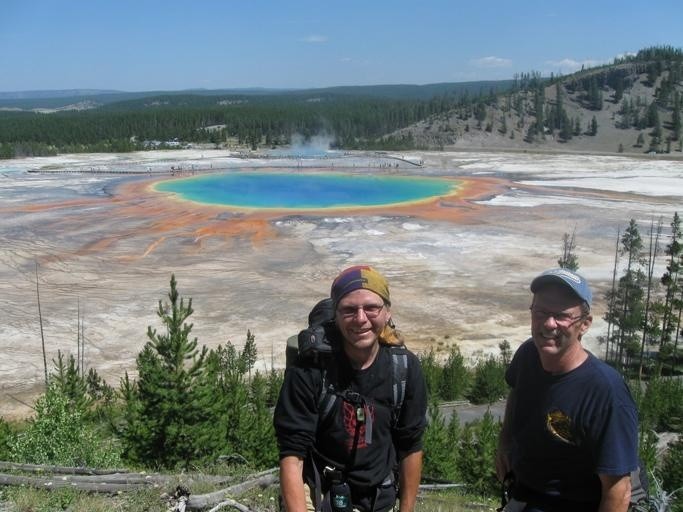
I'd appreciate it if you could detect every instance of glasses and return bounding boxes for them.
[530,305,584,327]
[336,303,385,319]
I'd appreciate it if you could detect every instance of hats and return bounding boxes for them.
[530,268,593,311]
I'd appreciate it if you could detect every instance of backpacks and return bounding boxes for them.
[285,298,410,436]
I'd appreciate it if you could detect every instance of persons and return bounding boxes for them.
[494,268,639,510]
[272,264,429,512]
